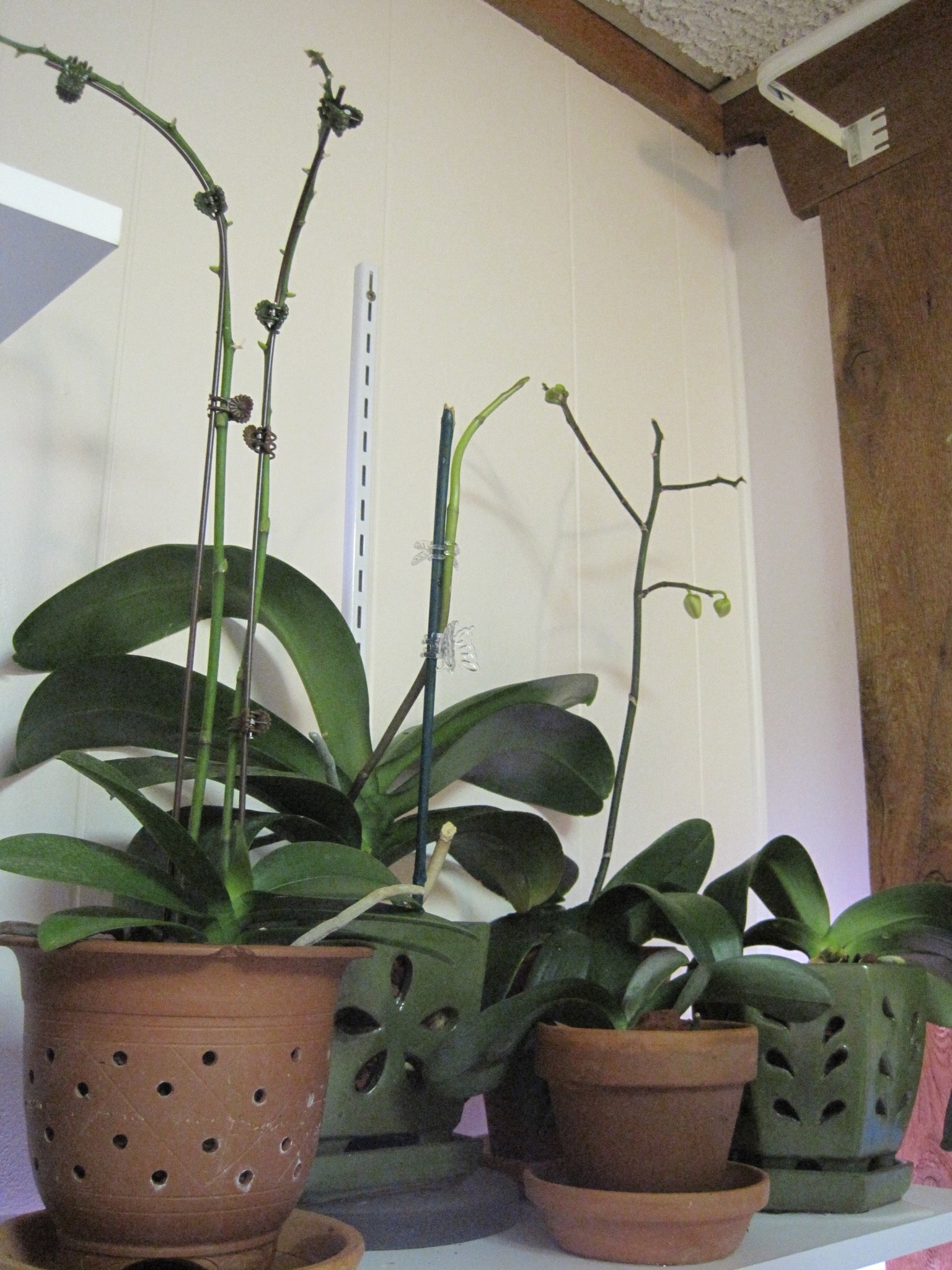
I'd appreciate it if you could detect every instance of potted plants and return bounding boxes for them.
[0,35,376,1269]
[17,378,621,1146]
[681,833,952,1213]
[468,392,750,1156]
[419,872,793,1264]
